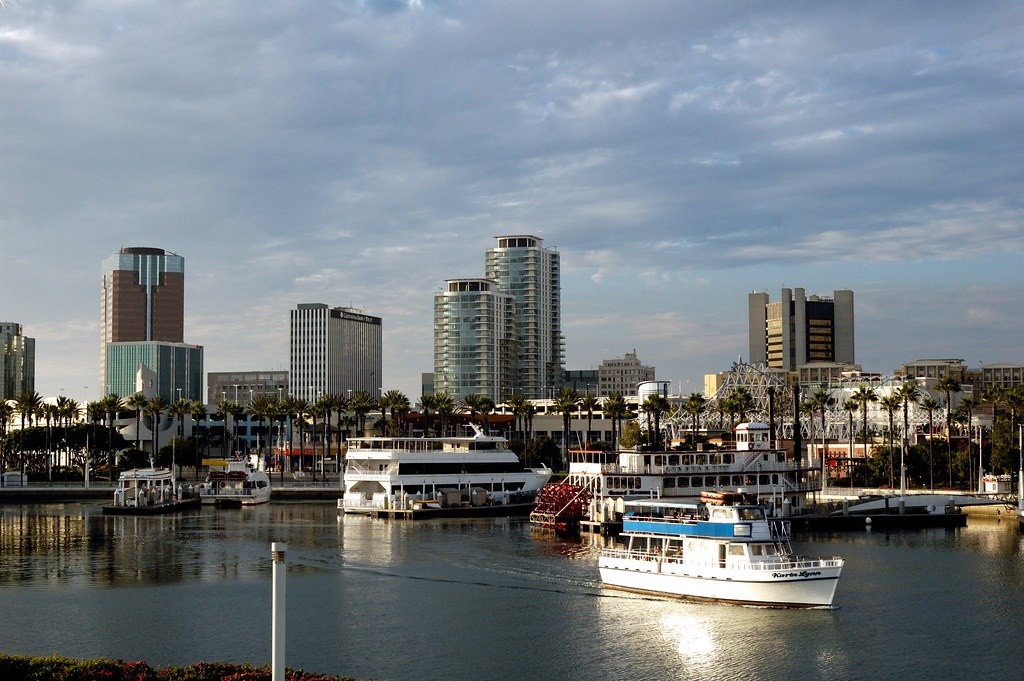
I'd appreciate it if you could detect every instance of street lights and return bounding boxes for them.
[60,379,681,412]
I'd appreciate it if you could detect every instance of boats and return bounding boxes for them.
[102,457,199,514]
[194,450,271,505]
[337,422,553,521]
[598,482,844,609]
[525,422,824,538]
